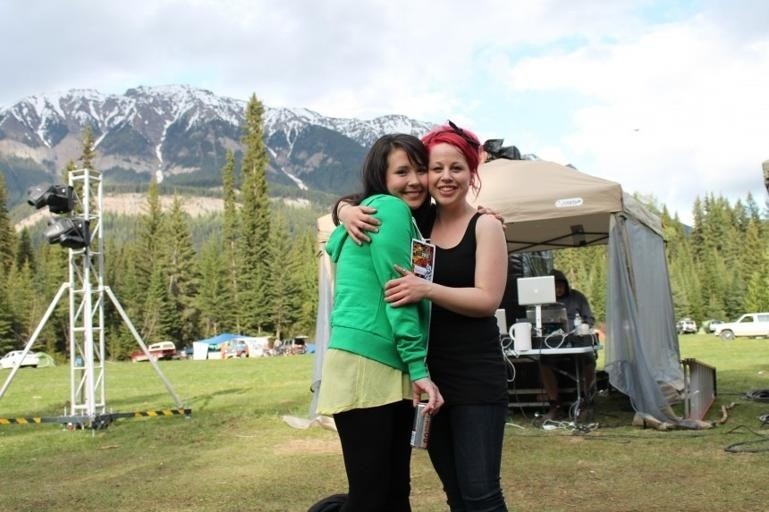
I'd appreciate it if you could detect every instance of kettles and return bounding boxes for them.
[509,321,533,352]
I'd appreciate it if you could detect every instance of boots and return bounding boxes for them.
[547,400,566,419]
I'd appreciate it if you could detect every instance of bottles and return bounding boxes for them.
[574,313,583,335]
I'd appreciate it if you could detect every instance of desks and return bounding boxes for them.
[503,344,604,423]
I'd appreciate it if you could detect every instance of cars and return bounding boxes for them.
[709,320,724,332]
[1,350,38,369]
[714,312,768,339]
[676,320,696,334]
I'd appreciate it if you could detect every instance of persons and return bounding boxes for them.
[539,271,599,420]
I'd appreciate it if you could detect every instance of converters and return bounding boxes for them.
[543,424,555,430]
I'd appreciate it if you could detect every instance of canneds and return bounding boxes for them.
[409,403,431,449]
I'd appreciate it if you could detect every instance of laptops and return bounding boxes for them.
[516,275,563,304]
[495,309,508,335]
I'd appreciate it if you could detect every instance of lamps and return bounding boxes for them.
[27,182,90,248]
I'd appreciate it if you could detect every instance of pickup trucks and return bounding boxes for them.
[134,339,177,362]
